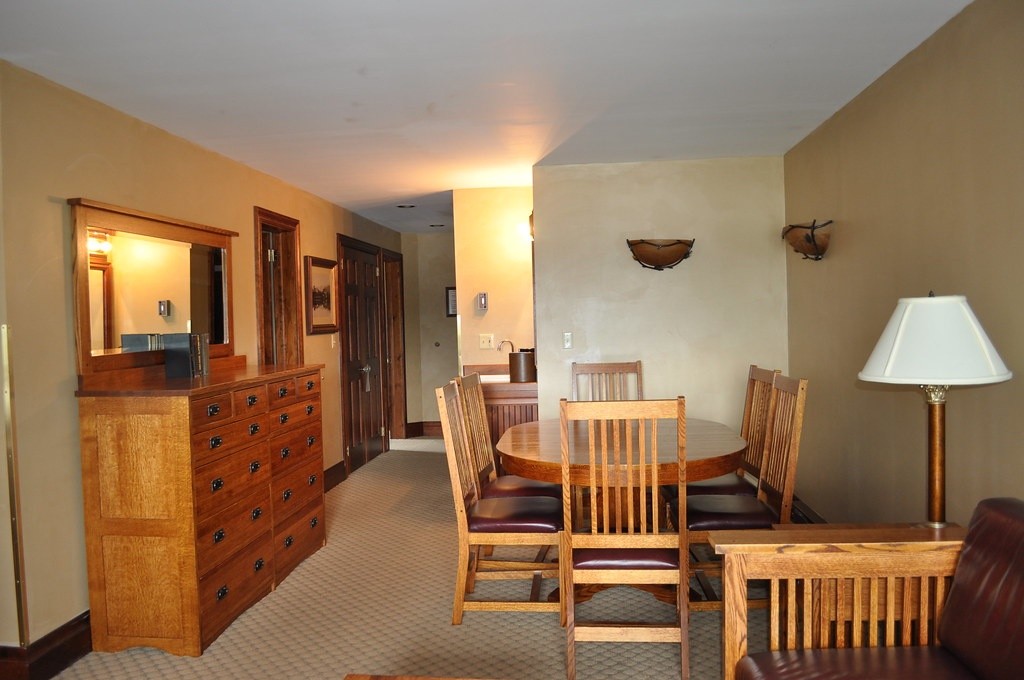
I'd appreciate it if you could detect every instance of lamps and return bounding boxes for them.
[781,218,833,261]
[856,290,1013,530]
[625,238,695,271]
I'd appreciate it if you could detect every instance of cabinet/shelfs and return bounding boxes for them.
[74,364,327,658]
[463,363,538,476]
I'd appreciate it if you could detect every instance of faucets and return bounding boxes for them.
[496,339,515,353]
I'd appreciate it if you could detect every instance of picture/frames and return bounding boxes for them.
[445,287,457,317]
[304,255,340,335]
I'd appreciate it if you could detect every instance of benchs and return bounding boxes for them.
[705,498,1024,680]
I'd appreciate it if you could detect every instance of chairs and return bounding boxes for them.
[660,365,809,644]
[572,360,644,531]
[435,370,573,627]
[559,396,690,680]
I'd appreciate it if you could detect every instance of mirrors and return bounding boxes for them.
[66,197,240,374]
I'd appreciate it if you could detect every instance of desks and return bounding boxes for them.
[772,518,966,651]
[495,418,749,606]
